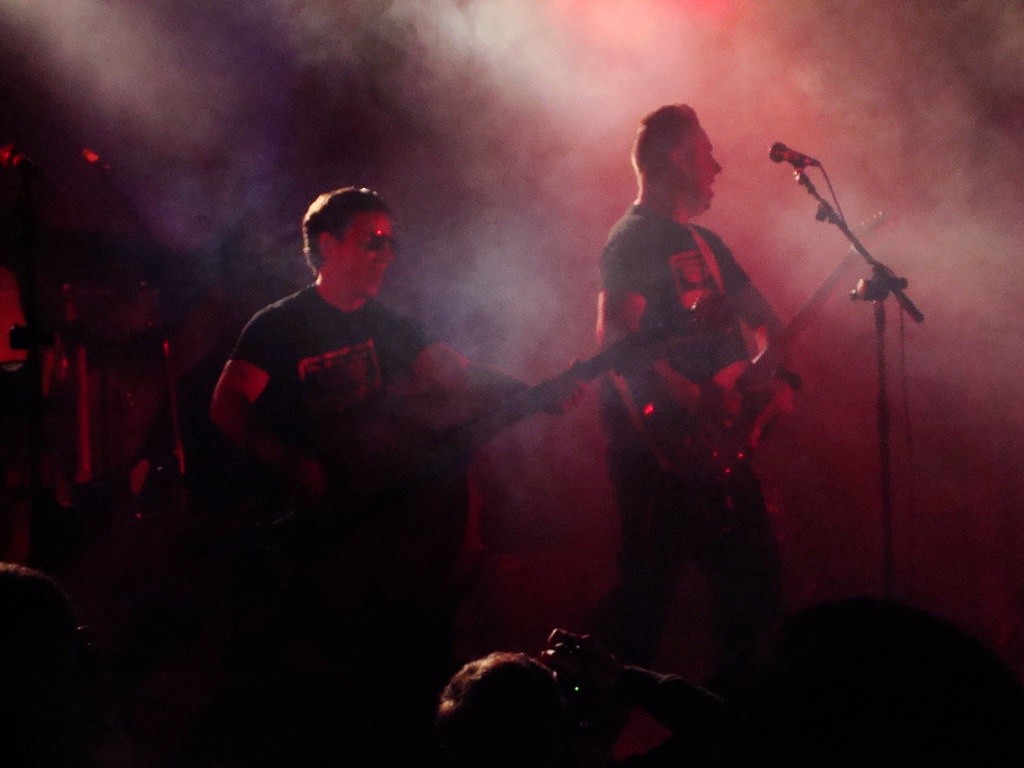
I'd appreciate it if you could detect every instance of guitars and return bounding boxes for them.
[693,209,886,453]
[275,319,710,600]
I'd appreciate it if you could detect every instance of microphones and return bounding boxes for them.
[769,142,821,166]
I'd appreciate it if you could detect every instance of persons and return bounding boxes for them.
[570,104,803,760]
[435,628,736,767]
[0,562,102,768]
[209,184,483,768]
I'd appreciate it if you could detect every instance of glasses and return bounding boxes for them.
[360,231,396,252]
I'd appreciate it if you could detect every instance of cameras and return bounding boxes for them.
[537,643,587,694]
